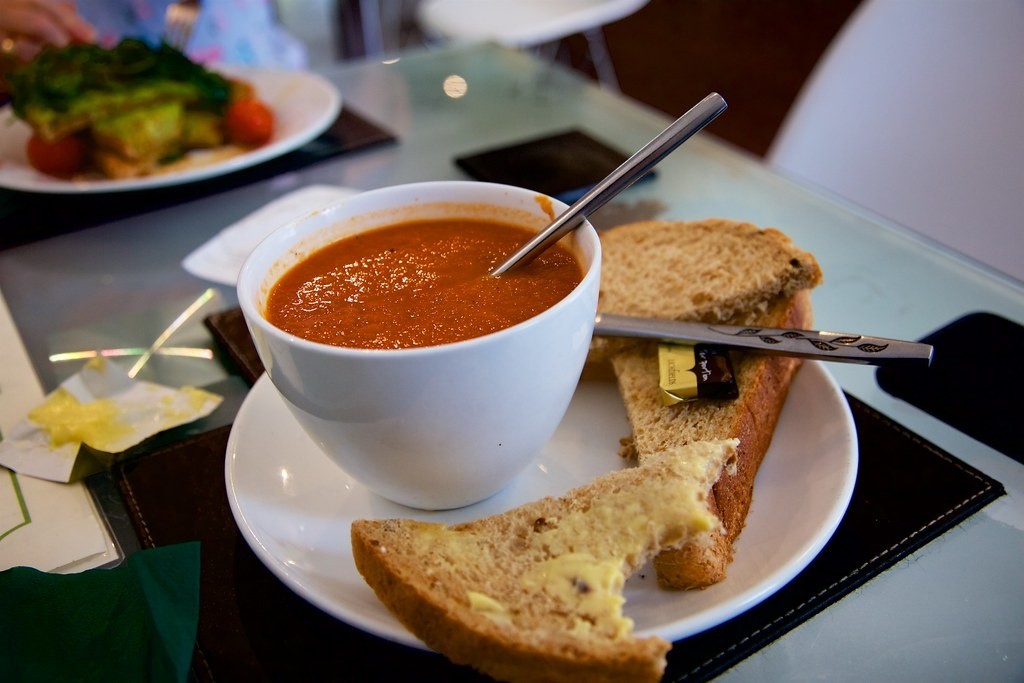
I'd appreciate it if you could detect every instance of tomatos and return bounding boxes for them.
[225,100,273,148]
[27,133,90,179]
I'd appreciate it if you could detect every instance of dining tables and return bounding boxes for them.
[0,38,1024,683]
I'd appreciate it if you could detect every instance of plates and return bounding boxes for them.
[225,357,860,652]
[0,68,344,191]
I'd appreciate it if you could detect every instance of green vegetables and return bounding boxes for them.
[6,34,233,124]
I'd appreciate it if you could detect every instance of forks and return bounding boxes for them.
[160,1,201,55]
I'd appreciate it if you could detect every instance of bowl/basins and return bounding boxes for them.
[236,182,601,510]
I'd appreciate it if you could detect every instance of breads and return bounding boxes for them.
[350,437,734,683]
[607,288,812,592]
[585,218,824,362]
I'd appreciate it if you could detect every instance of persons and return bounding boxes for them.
[0,1,309,106]
